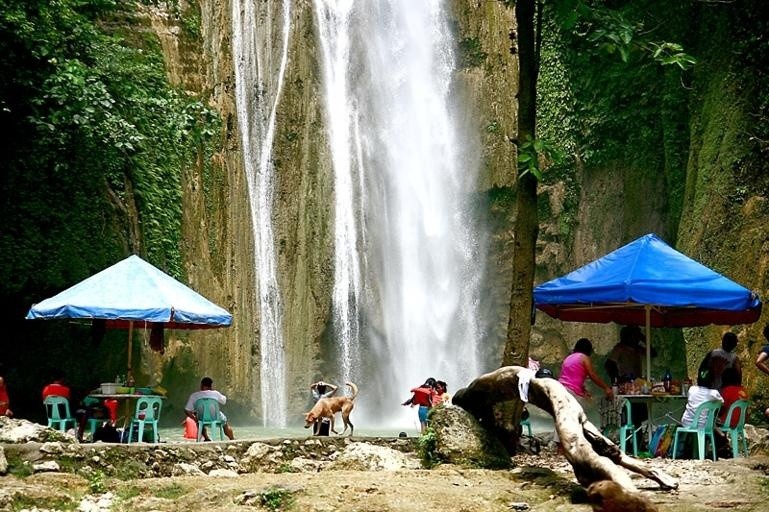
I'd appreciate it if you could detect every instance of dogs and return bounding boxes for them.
[302,382,358,436]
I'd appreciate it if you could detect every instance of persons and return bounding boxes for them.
[713,369,749,450]
[310,380,338,436]
[605,324,657,451]
[553,337,616,457]
[434,381,452,406]
[680,367,725,438]
[185,377,235,441]
[755,324,769,416]
[411,384,434,436]
[42,372,86,440]
[424,377,437,390]
[0,377,13,416]
[519,406,531,437]
[698,332,742,387]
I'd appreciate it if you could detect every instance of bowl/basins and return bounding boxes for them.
[117,386,135,394]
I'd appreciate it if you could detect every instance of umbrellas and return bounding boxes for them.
[532,235,763,451]
[24,253,232,429]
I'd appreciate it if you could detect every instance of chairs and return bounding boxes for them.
[81,393,170,445]
[44,395,79,438]
[716,397,754,460]
[598,395,641,457]
[194,396,232,445]
[672,399,726,462]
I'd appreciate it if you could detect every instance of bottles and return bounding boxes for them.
[612,375,663,394]
[664,368,672,392]
[115,375,135,388]
[674,384,681,392]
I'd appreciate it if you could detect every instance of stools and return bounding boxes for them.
[519,419,533,438]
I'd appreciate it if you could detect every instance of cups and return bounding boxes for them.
[682,385,688,395]
